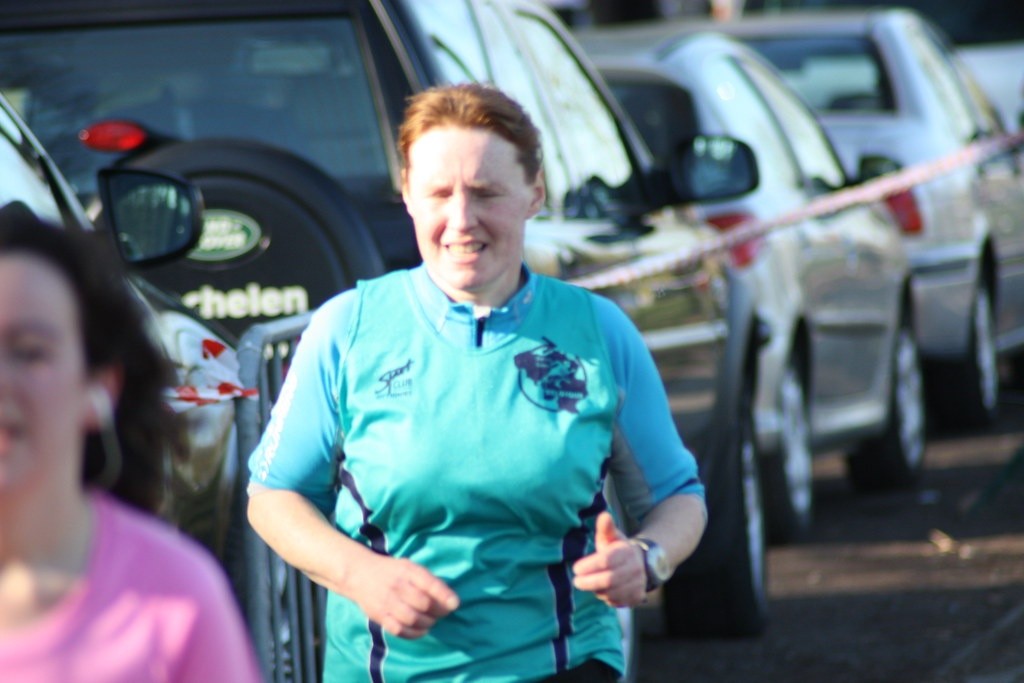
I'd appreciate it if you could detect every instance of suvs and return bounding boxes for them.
[0,0,774,683]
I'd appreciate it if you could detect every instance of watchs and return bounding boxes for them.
[632,538,672,592]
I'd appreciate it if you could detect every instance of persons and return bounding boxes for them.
[0,201,263,683]
[248,81,708,683]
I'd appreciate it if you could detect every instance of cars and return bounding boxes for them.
[578,9,1024,540]
